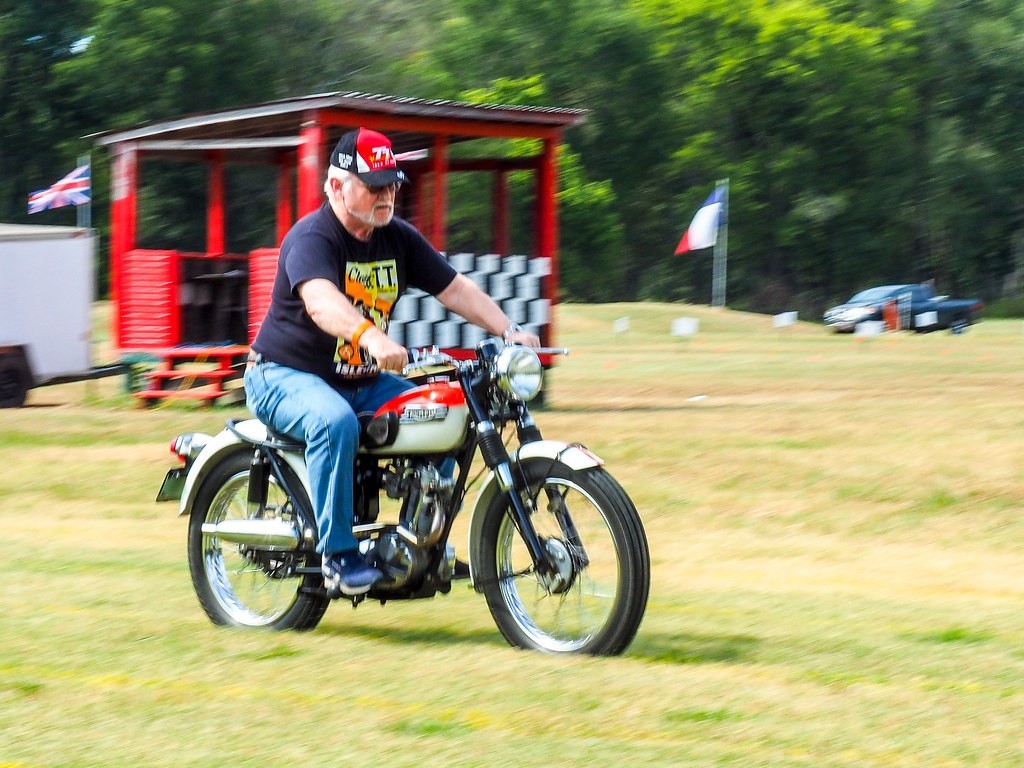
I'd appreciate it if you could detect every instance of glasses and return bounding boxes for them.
[365,180,401,195]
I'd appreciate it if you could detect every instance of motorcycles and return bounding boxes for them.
[154,334,652,658]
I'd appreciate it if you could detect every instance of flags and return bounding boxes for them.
[672,185,727,256]
[27,165,93,215]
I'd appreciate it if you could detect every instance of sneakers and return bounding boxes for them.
[321,552,383,595]
[451,558,468,583]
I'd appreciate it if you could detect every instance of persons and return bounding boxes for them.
[242,125,542,595]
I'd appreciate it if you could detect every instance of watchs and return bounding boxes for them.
[502,323,523,339]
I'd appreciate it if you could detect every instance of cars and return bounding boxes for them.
[821,283,984,333]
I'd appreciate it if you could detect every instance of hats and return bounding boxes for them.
[330,126,409,186]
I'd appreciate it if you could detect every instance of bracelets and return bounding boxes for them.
[337,319,376,366]
